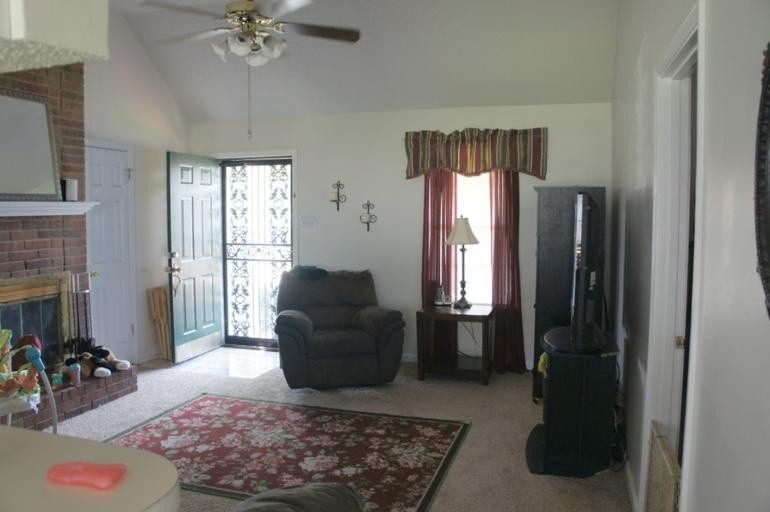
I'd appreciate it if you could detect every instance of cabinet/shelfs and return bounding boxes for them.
[525,185,619,478]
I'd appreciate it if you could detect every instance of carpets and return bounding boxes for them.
[99,391,472,512]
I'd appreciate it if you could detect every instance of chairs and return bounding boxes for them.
[0,341,40,426]
[274,269,406,388]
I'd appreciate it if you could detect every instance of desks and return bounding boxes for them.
[0,425,179,512]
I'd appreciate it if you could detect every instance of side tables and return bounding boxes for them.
[416,303,496,386]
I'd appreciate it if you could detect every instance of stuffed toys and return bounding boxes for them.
[7,336,130,393]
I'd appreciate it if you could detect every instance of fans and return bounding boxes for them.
[138,0,360,48]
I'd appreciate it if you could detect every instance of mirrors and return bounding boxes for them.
[0,87,63,201]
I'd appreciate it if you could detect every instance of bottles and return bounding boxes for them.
[69,363,80,385]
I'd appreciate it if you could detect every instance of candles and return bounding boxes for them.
[361,213,370,222]
[329,190,337,200]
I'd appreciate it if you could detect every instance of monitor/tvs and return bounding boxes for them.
[562,190,609,355]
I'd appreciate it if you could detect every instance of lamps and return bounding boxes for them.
[213,33,287,68]
[445,215,479,308]
